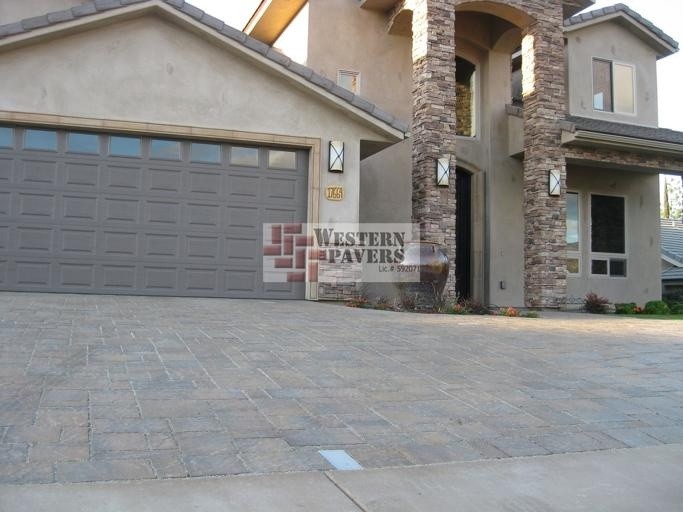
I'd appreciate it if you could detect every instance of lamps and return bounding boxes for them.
[549,169,561,196]
[437,158,450,186]
[329,141,345,172]
